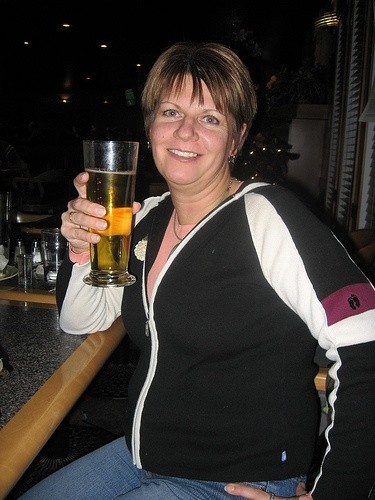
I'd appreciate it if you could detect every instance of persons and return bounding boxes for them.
[7,39,375,500]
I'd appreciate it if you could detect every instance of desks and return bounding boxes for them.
[0,201,127,500]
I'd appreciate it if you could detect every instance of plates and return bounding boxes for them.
[0,265,18,281]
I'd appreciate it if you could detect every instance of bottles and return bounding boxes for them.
[30,238,42,267]
[14,239,26,264]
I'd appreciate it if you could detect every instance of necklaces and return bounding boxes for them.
[173,174,237,240]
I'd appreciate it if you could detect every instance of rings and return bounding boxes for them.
[68,211,79,224]
[267,491,275,499]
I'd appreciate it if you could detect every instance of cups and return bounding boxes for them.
[17,254,33,292]
[82,139,140,287]
[41,228,69,287]
